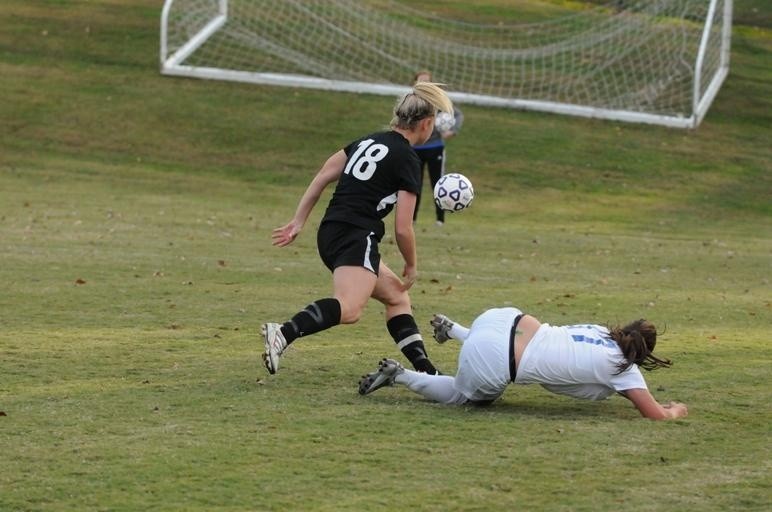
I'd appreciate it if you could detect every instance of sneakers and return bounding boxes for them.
[359,358,404,395]
[262,323,287,374]
[430,314,454,344]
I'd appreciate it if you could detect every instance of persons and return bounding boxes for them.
[358,307,688,419]
[412,70,465,224]
[260,81,441,376]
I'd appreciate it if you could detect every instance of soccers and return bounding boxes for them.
[434,112,456,133]
[434,173,474,212]
